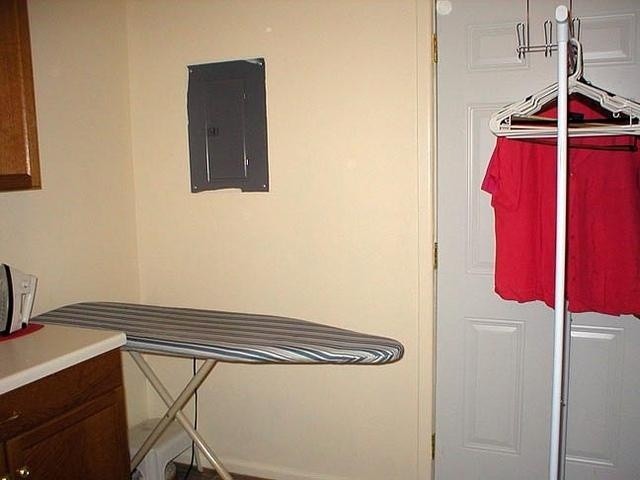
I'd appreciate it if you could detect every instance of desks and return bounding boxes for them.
[25,302,404,479]
[1,322,130,479]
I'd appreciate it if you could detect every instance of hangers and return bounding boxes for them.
[488,36,639,142]
